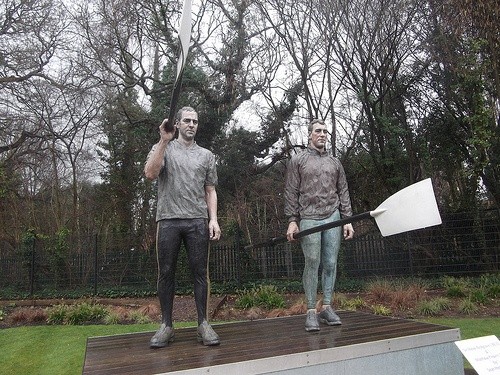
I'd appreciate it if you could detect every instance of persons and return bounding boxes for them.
[144,107,222,348]
[283,120,355,332]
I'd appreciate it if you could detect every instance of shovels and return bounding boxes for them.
[245,177,442,252]
[163,0,192,133]
